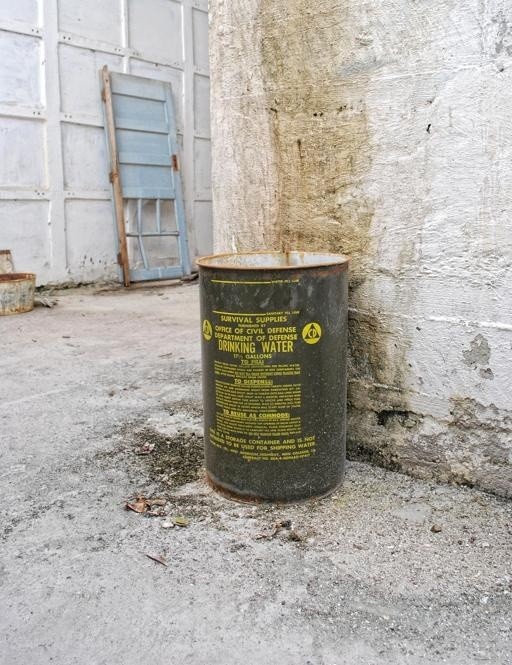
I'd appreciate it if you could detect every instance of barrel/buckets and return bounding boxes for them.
[194,249,351,506]
